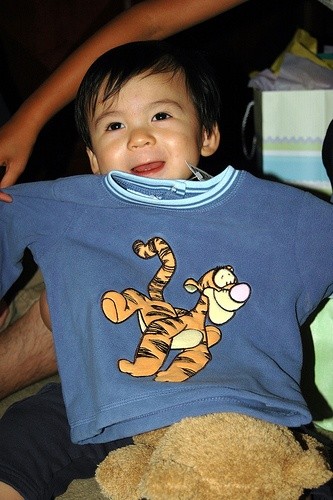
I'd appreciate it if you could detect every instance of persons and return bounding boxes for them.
[1,290,57,397]
[1,40,333,500]
[0,0,246,204]
